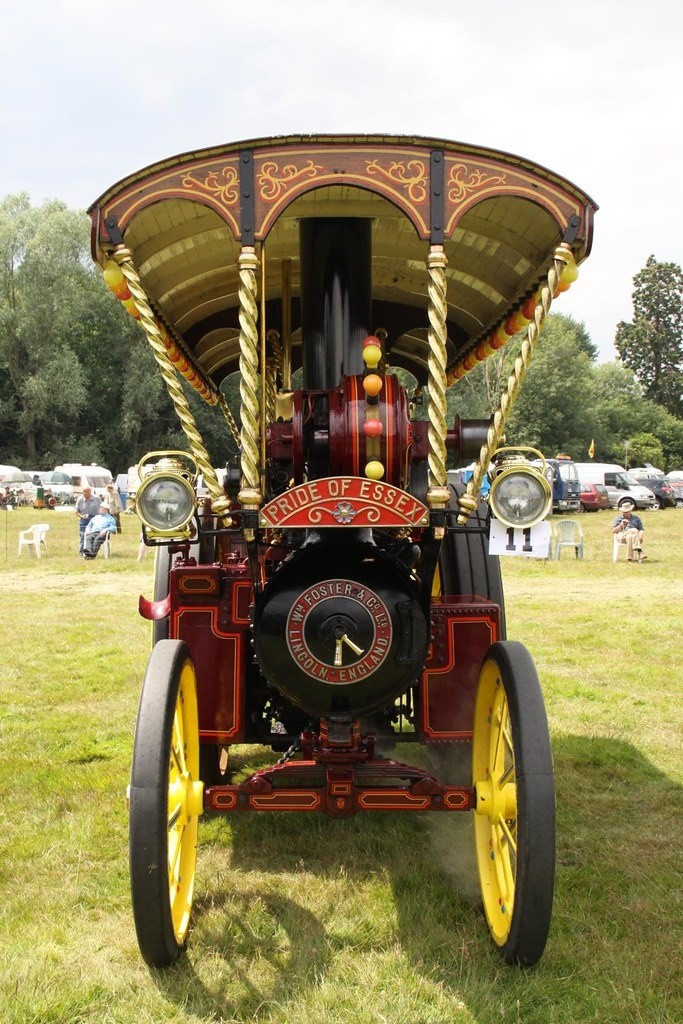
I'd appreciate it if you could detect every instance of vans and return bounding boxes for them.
[127,463,154,499]
[627,468,664,479]
[114,473,128,494]
[547,463,656,512]
[54,463,115,501]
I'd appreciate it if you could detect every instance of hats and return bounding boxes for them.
[619,502,634,513]
[99,502,110,510]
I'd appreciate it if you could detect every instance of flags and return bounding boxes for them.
[588,438,595,458]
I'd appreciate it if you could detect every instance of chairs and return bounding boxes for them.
[613,533,641,563]
[83,531,112,559]
[18,524,51,559]
[555,520,585,560]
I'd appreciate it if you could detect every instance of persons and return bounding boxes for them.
[124,499,137,514]
[107,485,124,534]
[3,487,26,507]
[83,502,117,560]
[612,502,647,563]
[75,487,103,558]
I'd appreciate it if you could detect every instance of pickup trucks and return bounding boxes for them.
[22,471,75,505]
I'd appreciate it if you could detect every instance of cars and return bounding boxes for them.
[574,483,610,514]
[534,458,580,514]
[663,471,683,499]
[84,132,602,967]
[635,479,676,510]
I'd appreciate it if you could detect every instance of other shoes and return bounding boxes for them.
[639,555,647,560]
[83,549,96,560]
[628,559,631,563]
